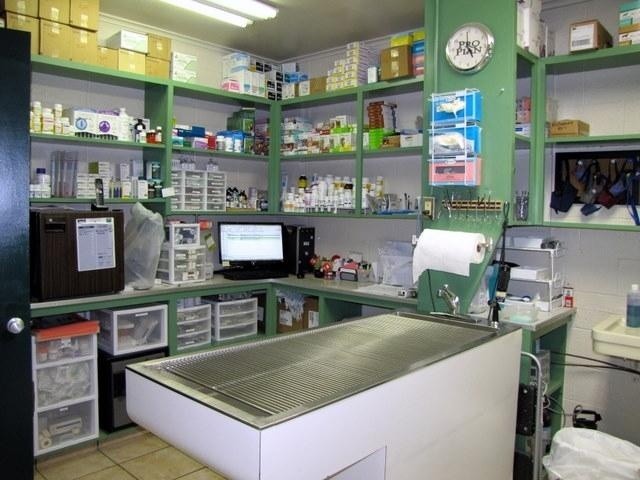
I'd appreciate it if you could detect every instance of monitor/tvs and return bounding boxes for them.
[219,222,287,280]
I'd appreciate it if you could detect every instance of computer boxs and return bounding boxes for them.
[283,223,315,275]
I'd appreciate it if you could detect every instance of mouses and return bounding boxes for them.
[297,273,305,280]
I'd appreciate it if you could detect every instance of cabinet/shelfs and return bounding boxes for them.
[29,43,639,480]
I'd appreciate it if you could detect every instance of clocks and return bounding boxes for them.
[443,22,495,75]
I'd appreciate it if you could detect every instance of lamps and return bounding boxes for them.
[161,0,280,28]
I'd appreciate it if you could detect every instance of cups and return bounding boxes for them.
[516,189,531,222]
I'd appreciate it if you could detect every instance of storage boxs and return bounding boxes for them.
[0,0,171,79]
[568,18,614,55]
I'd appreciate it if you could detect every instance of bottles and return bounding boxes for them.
[119,107,128,116]
[215,135,242,152]
[285,173,384,212]
[135,117,162,143]
[30,168,51,199]
[627,283,640,327]
